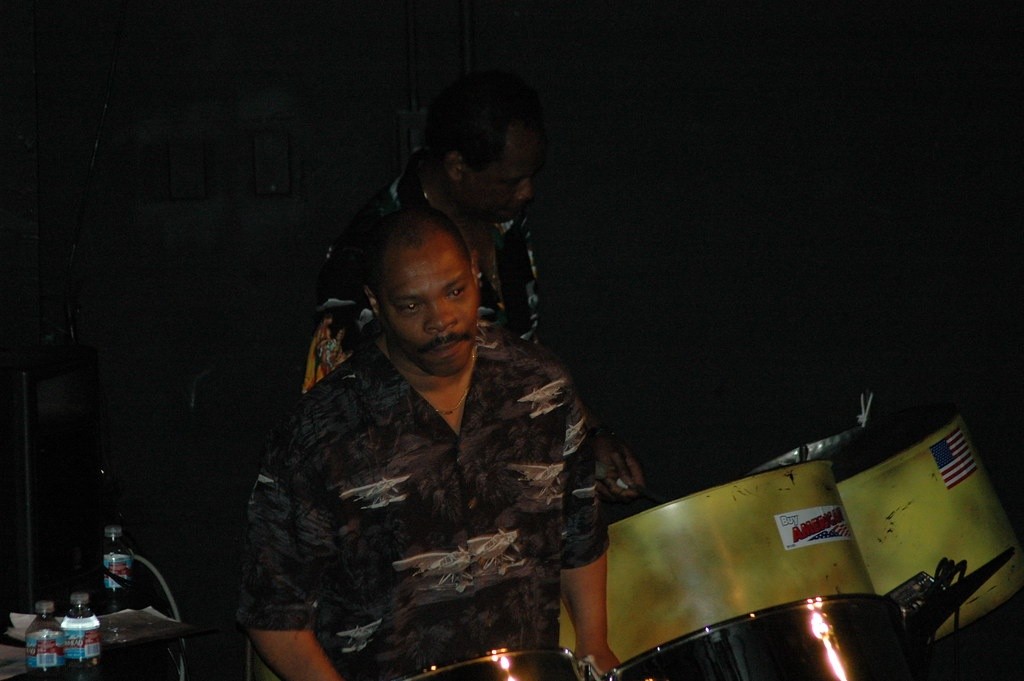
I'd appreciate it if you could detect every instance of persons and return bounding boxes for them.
[235,201,624,681]
[295,67,652,503]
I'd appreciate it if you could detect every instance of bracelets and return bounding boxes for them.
[584,421,617,443]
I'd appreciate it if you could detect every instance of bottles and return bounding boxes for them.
[101,523,134,613]
[59,592,101,681]
[23,601,64,681]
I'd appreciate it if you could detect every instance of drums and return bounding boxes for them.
[554,456,876,664]
[809,405,1022,644]
[611,593,928,679]
[403,647,583,680]
